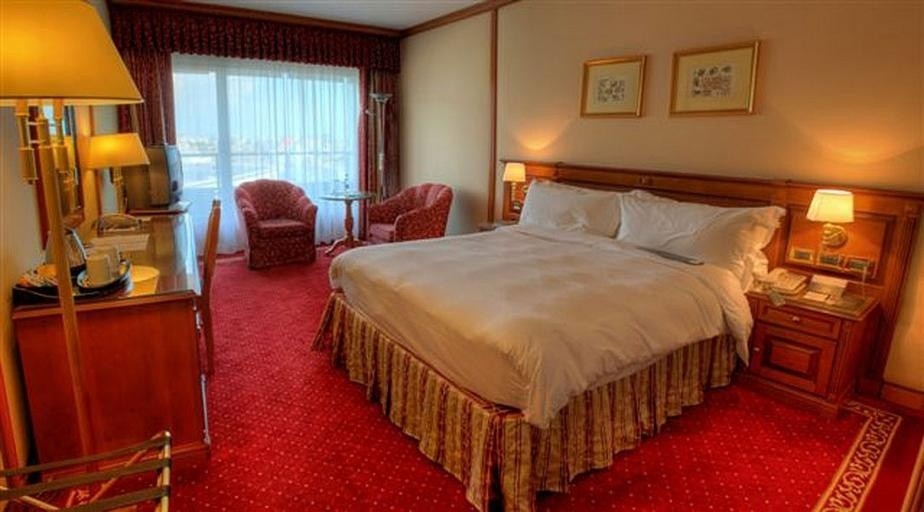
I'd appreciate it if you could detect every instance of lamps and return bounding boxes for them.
[804,188,853,250]
[499,159,525,212]
[83,130,148,230]
[3,0,148,509]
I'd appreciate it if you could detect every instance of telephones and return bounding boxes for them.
[763,267,808,295]
[104,212,137,228]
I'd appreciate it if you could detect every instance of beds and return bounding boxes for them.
[296,159,783,512]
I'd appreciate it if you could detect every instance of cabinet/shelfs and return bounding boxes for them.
[364,88,391,200]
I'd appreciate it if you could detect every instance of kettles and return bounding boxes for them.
[44,228,89,276]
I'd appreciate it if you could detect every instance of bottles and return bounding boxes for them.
[343,173,350,194]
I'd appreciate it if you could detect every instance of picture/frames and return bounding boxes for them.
[666,38,760,119]
[580,53,646,119]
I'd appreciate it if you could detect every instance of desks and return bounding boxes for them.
[13,212,209,491]
[320,193,373,254]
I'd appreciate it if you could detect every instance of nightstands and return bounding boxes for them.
[745,279,881,418]
[477,220,516,231]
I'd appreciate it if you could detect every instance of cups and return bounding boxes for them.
[96,245,120,271]
[85,254,110,285]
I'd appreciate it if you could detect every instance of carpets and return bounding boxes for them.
[4,236,924,512]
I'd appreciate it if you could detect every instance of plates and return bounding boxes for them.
[16,281,59,296]
[76,260,130,291]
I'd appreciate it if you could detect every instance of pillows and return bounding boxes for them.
[617,190,788,276]
[515,180,620,241]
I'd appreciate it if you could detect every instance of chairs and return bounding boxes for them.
[234,176,318,269]
[200,197,227,379]
[366,184,452,245]
